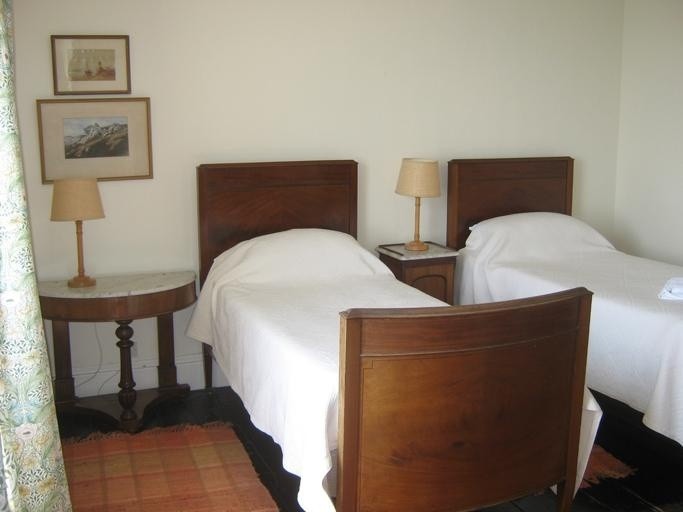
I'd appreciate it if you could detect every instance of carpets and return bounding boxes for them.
[580,444,635,487]
[62,421,280,511]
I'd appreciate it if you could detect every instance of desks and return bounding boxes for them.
[38,271,198,434]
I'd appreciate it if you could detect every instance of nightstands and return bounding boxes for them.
[375,241,459,305]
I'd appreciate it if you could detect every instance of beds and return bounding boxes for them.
[447,156,682,445]
[186,160,604,512]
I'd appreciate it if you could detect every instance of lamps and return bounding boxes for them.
[395,158,441,251]
[50,177,106,288]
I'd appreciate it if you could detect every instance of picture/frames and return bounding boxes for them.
[51,35,131,95]
[36,98,154,185]
